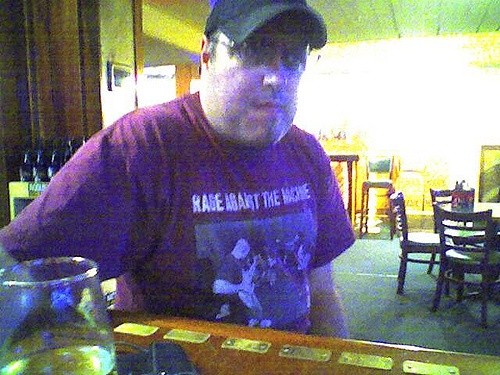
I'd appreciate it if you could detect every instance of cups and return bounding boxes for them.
[0,257,118,375]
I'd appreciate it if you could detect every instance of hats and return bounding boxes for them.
[205,1,326,49]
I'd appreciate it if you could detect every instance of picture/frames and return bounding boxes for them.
[108,63,134,93]
[478,144,500,203]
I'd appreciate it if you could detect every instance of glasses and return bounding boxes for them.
[217,43,306,75]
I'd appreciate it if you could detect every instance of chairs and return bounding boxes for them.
[427,189,460,274]
[390,192,452,296]
[433,204,500,328]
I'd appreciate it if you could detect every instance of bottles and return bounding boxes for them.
[18,135,89,182]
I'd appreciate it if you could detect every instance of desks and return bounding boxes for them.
[329,155,360,227]
[1,309,500,375]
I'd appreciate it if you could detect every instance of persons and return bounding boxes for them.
[0,0,358,339]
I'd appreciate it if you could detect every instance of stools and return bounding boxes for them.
[360,182,397,240]
